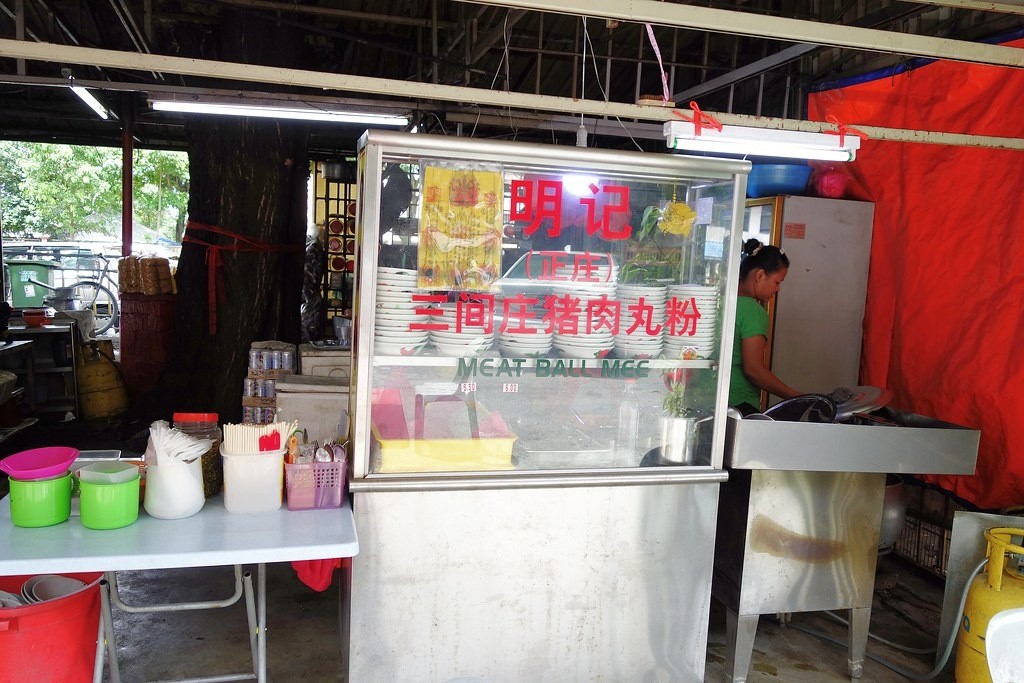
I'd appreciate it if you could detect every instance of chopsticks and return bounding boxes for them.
[223,420,300,453]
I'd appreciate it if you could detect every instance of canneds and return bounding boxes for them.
[243,350,293,427]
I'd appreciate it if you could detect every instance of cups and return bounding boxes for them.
[336,325,351,346]
[145,456,205,520]
[8,469,73,528]
[79,469,143,530]
[220,441,288,514]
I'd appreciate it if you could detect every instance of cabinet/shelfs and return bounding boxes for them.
[7,319,81,420]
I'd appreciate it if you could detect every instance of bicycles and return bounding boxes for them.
[69,250,123,335]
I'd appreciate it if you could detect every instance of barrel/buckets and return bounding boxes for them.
[657,415,714,465]
[0,572,112,683]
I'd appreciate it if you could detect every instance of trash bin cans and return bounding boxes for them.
[3,259,61,307]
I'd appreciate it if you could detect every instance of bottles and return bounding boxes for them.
[172,412,222,498]
[617,377,641,465]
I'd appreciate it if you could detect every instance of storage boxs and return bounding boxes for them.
[274,374,350,445]
[370,400,517,473]
[0,386,25,428]
[297,343,351,377]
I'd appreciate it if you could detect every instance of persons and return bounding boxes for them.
[728,238,804,418]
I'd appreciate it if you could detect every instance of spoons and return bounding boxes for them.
[316,443,346,508]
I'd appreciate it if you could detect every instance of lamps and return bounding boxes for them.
[660,121,861,163]
[146,91,413,126]
[60,68,110,120]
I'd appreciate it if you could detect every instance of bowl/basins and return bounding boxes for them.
[664,284,718,360]
[374,266,430,356]
[22,309,55,326]
[429,302,496,357]
[497,320,552,358]
[0,575,88,608]
[553,263,620,358]
[615,282,668,359]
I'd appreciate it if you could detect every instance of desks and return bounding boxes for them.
[0,457,359,683]
